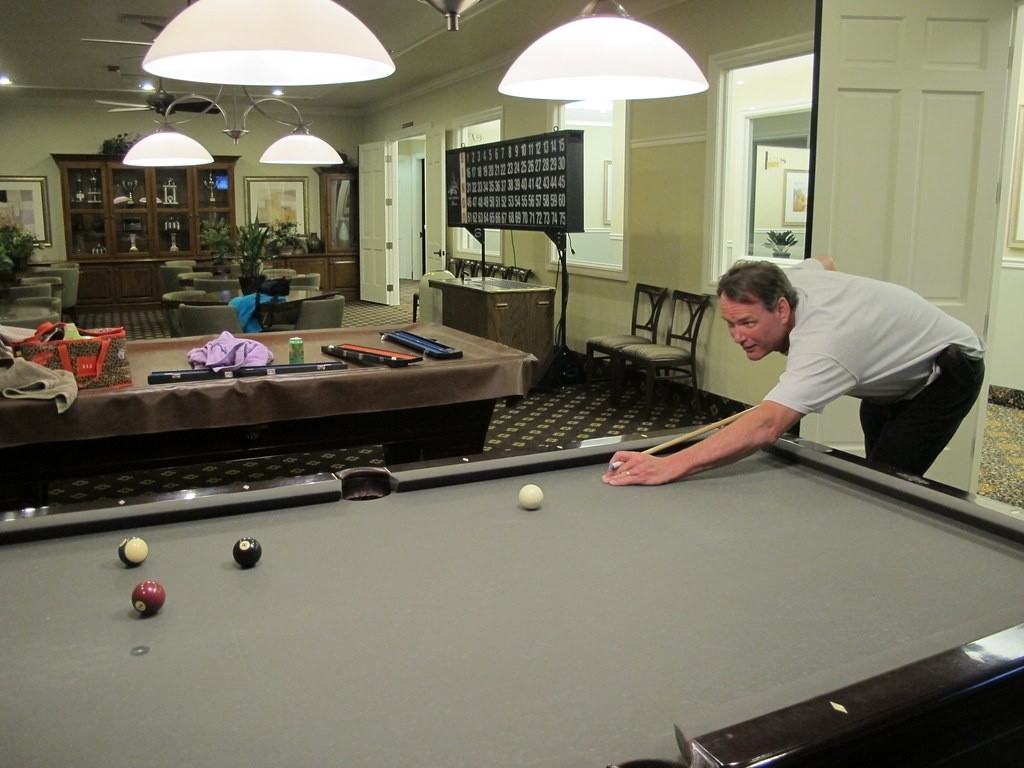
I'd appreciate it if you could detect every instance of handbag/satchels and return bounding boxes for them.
[12,320,132,391]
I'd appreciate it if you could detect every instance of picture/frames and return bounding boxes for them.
[0,176,53,248]
[242,175,310,238]
[780,169,810,226]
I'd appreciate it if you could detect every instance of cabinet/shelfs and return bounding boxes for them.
[278,252,332,291]
[49,153,242,311]
[312,167,360,296]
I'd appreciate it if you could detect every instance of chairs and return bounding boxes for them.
[584,283,669,406]
[0,262,81,330]
[155,260,346,338]
[619,289,712,418]
[413,258,532,322]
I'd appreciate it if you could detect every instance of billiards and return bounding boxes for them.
[232,537,261,566]
[518,484,544,509]
[131,581,165,615]
[118,536,150,566]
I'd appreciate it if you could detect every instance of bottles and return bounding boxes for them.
[63,323,81,339]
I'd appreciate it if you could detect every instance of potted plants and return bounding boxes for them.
[0,241,17,285]
[761,230,799,259]
[199,215,235,276]
[234,206,272,297]
[0,222,46,272]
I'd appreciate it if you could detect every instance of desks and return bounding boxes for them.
[162,290,341,328]
[427,275,556,390]
[0,421,1024,768]
[0,319,538,508]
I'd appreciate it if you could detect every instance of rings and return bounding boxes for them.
[624,471,631,476]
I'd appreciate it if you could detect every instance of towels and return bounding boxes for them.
[227,292,287,332]
[187,331,274,373]
[0,340,79,414]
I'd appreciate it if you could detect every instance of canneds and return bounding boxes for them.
[288,336,305,364]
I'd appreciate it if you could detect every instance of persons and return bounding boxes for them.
[602,258,986,486]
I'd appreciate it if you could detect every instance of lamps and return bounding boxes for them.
[241,96,344,165]
[119,93,228,169]
[419,0,480,32]
[140,0,396,88]
[497,0,710,103]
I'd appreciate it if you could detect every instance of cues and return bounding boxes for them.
[609,404,761,471]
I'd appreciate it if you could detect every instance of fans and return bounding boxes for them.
[92,76,222,116]
